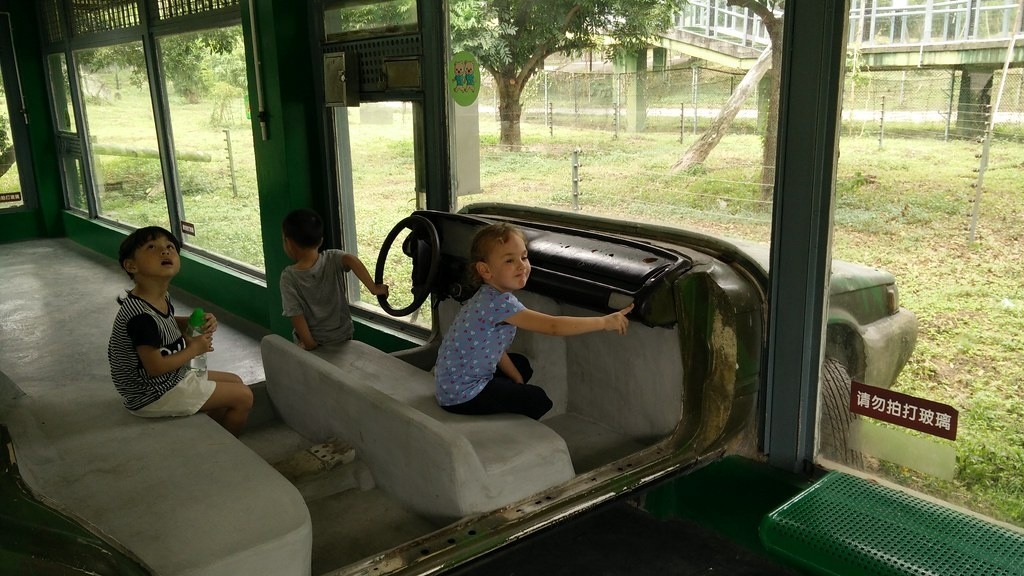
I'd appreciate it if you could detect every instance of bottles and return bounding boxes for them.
[184,308,208,377]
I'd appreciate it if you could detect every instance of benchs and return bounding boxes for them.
[0,371,313,576]
[260,334,576,530]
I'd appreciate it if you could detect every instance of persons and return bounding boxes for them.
[434,222,634,421]
[279,208,389,351]
[107,226,253,439]
